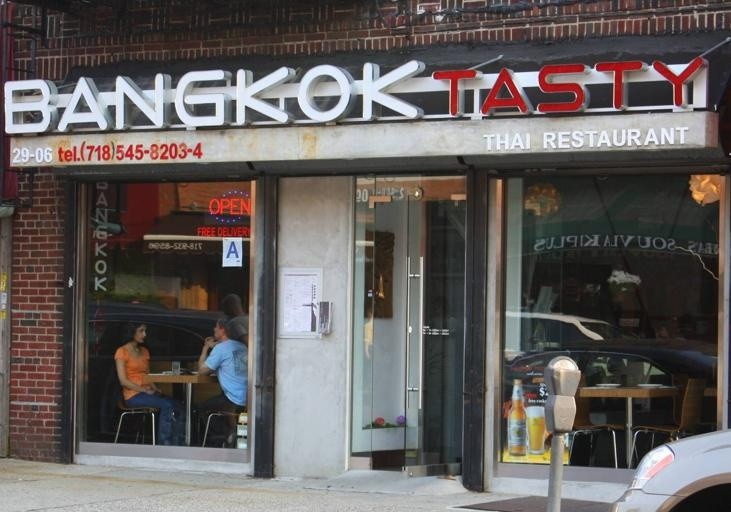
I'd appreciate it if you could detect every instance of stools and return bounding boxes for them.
[199,411,239,447]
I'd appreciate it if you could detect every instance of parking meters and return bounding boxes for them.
[539,356,581,511]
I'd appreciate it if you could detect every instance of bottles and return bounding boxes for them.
[507,378,528,455]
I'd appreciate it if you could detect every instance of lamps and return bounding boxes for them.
[690,175,722,207]
[526,184,562,219]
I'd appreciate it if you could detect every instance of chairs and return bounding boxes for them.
[567,375,641,469]
[625,378,705,471]
[113,374,158,445]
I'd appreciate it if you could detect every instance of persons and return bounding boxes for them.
[114,317,185,446]
[195,317,248,449]
[221,293,248,346]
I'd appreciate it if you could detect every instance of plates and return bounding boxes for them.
[596,383,621,387]
[638,384,663,388]
[192,372,199,374]
[163,371,183,374]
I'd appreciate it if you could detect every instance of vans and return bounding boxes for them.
[445,307,635,386]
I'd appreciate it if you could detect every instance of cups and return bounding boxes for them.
[171,361,180,376]
[525,406,550,455]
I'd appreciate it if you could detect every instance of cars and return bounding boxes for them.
[601,424,731,512]
[498,339,717,454]
[88,301,164,314]
[90,310,233,438]
